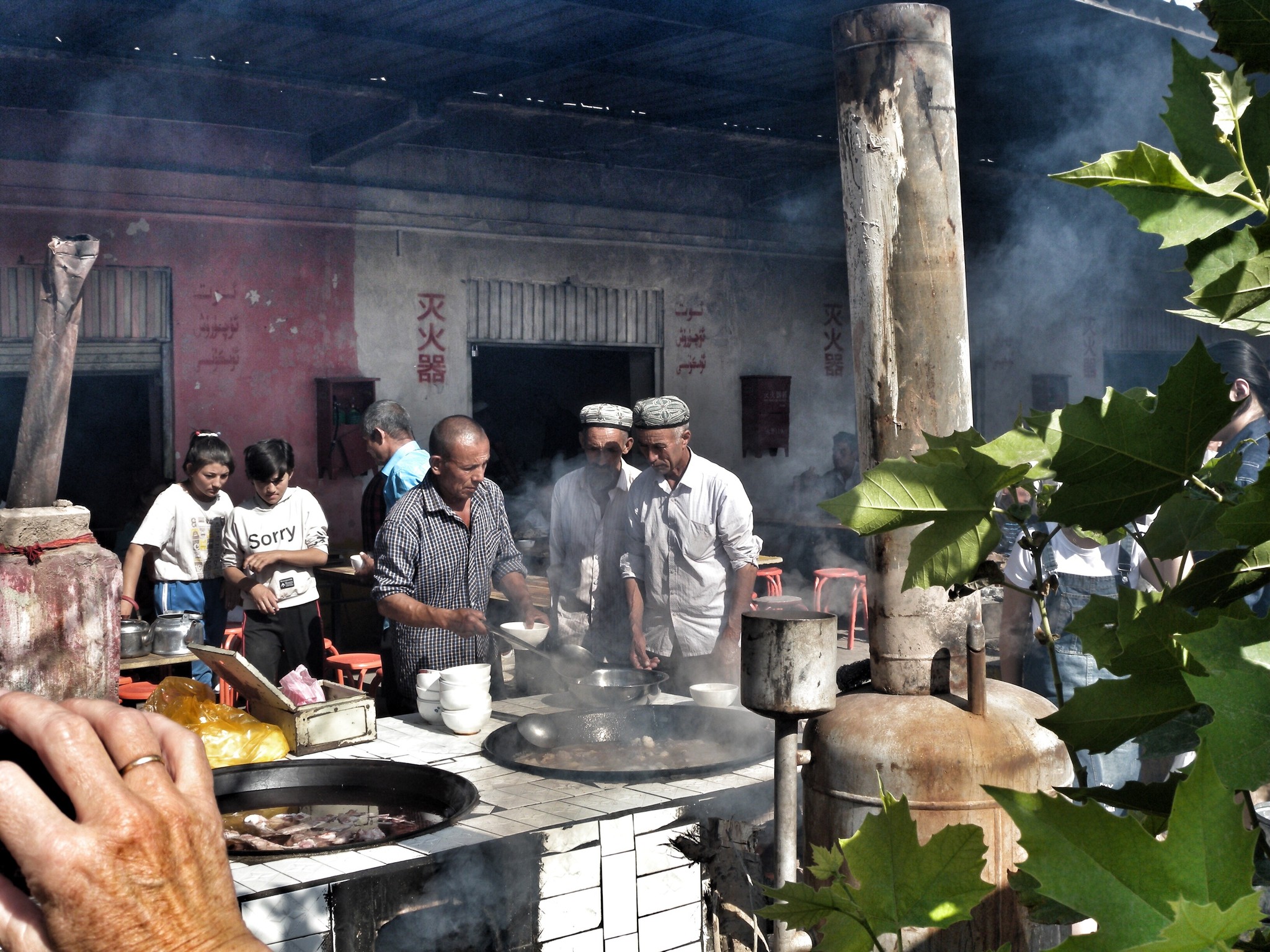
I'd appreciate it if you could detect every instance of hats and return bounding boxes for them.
[633,394,691,430]
[580,402,633,432]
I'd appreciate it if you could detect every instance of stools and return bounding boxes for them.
[813,568,861,615]
[749,590,759,612]
[324,651,384,692]
[324,638,355,686]
[846,574,869,649]
[751,594,809,612]
[366,668,385,704]
[217,621,245,706]
[756,565,784,610]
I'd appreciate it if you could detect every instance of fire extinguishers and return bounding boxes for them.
[347,402,361,424]
[329,395,346,455]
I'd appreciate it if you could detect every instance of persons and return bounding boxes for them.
[1147,339,1270,630]
[372,413,552,720]
[620,395,759,707]
[995,469,1196,817]
[115,428,235,691]
[0,689,274,951]
[359,398,436,696]
[516,483,557,537]
[546,403,644,669]
[222,437,331,708]
[549,436,587,481]
[995,485,1040,556]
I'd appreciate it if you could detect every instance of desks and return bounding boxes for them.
[757,553,784,565]
[315,564,549,704]
[751,508,852,530]
[118,645,203,687]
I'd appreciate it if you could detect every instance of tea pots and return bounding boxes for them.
[119,595,157,659]
[150,610,204,656]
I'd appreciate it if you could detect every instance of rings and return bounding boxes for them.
[363,556,371,560]
[118,754,165,777]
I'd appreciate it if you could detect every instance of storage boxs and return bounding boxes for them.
[188,642,378,758]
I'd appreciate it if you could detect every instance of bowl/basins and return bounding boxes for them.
[518,539,535,550]
[350,554,370,571]
[499,621,550,650]
[689,683,739,707]
[557,666,670,708]
[415,663,493,735]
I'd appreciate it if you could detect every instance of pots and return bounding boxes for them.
[484,703,776,782]
[211,758,479,866]
